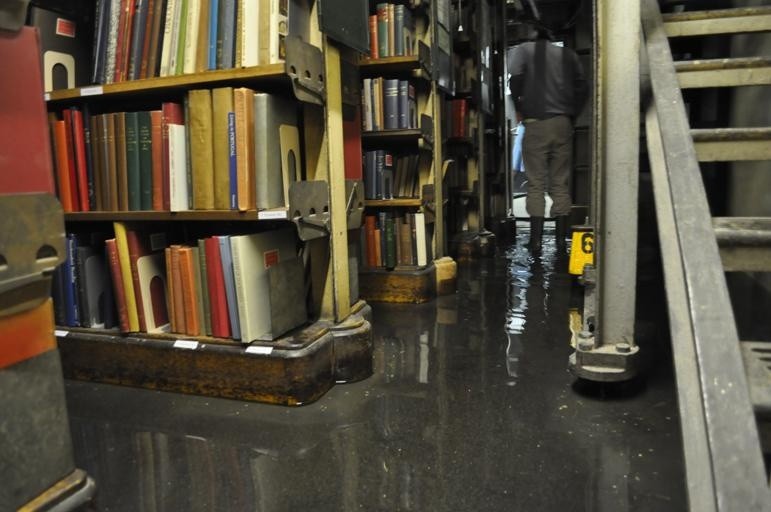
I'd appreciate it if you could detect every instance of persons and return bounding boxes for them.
[518,251,577,421]
[502,20,591,258]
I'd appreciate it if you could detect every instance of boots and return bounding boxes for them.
[555,215,568,247]
[523,218,544,252]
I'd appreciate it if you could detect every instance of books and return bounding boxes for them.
[368,2,414,59]
[360,150,420,199]
[51,86,297,212]
[362,77,418,131]
[54,221,296,343]
[363,210,427,269]
[437,6,479,230]
[90,0,289,85]
